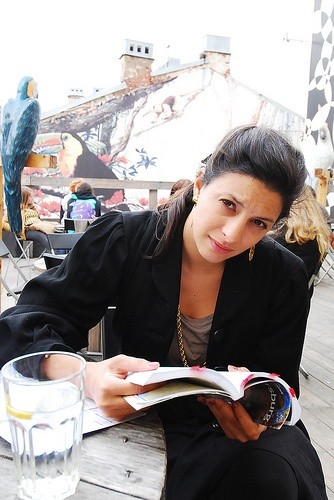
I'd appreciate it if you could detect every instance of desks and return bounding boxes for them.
[0,405,167,500]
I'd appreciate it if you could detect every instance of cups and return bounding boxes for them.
[1,351,86,500]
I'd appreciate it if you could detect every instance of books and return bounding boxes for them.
[125,363,303,431]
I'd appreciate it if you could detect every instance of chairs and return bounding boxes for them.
[0,218,94,304]
[298,242,334,379]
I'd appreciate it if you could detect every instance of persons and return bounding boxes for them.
[55,177,103,240]
[0,126,327,500]
[170,178,192,196]
[1,187,54,258]
[265,179,333,300]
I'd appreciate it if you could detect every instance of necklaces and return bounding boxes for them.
[177,303,208,368]
